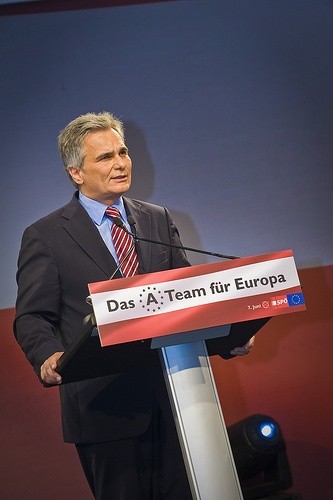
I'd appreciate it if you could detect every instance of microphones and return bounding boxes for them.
[108,216,139,279]
[114,217,239,259]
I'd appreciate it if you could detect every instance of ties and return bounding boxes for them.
[105,206,142,279]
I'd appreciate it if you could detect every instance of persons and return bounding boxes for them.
[12,109,256,500]
